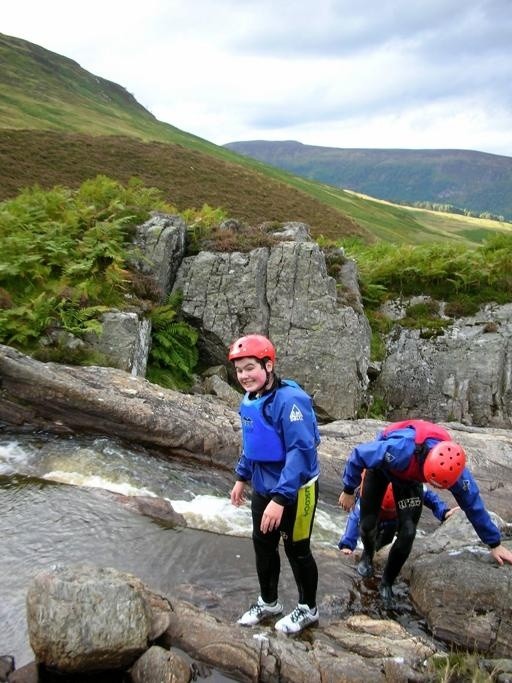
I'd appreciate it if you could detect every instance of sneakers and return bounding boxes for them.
[357,548,374,578]
[379,581,392,608]
[274,603,320,634]
[239,596,283,626]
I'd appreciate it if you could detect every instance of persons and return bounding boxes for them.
[337,467,461,557]
[338,419,511,610]
[228,332,322,638]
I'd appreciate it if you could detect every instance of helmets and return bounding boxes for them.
[228,334,275,365]
[381,481,398,521]
[423,441,466,490]
